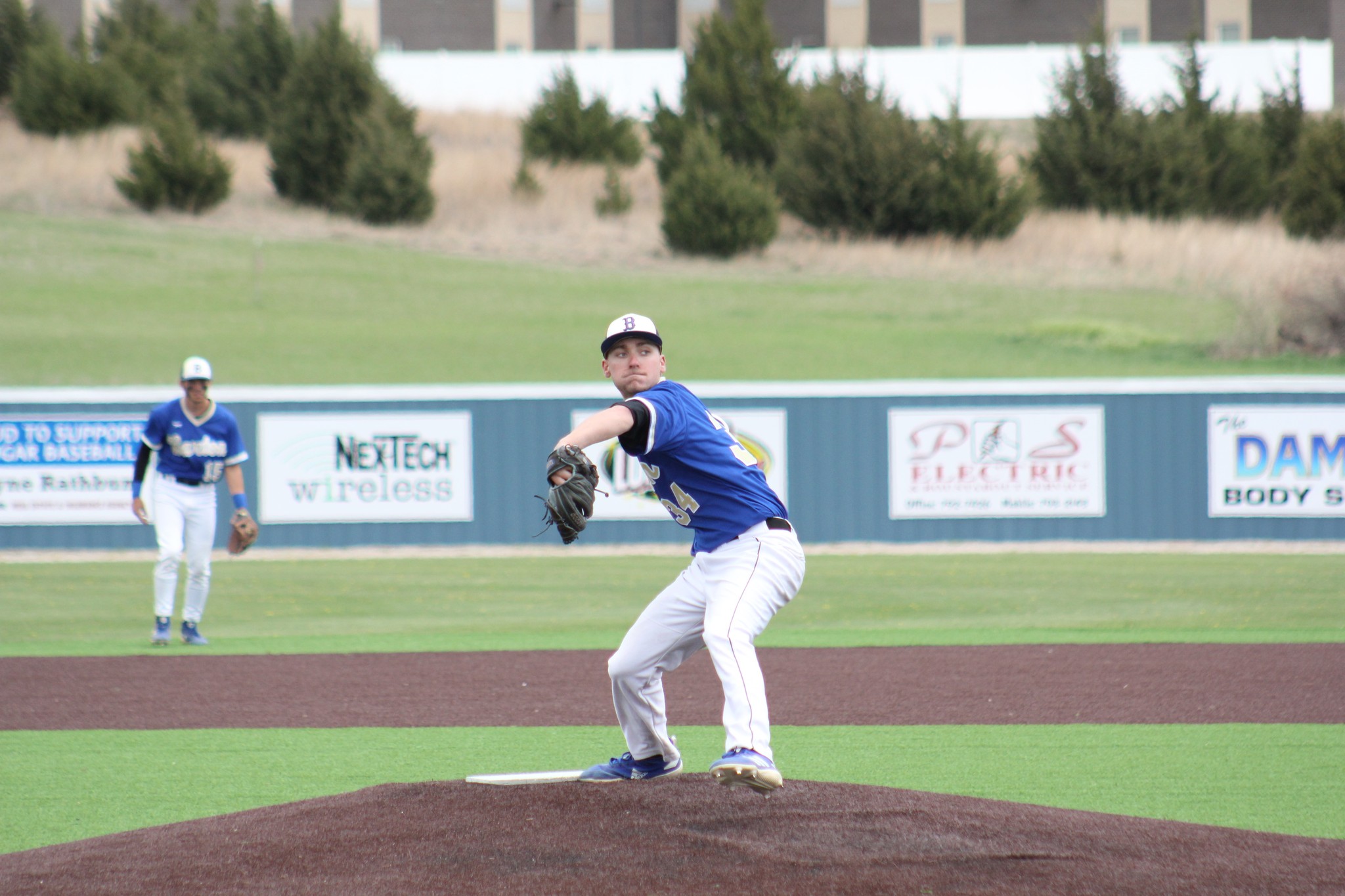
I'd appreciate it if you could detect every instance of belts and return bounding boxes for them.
[177,477,200,486]
[730,517,792,542]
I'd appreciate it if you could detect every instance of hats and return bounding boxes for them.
[181,356,213,382]
[600,312,663,359]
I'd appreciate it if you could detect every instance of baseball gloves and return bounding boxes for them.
[227,510,259,555]
[543,446,599,547]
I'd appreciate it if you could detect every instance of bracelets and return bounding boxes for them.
[232,493,248,509]
[131,481,142,498]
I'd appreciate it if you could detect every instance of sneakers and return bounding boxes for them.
[179,620,208,647]
[578,752,683,782]
[149,615,171,647]
[710,747,783,799]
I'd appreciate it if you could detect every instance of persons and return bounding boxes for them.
[531,311,807,799]
[130,354,260,648]
